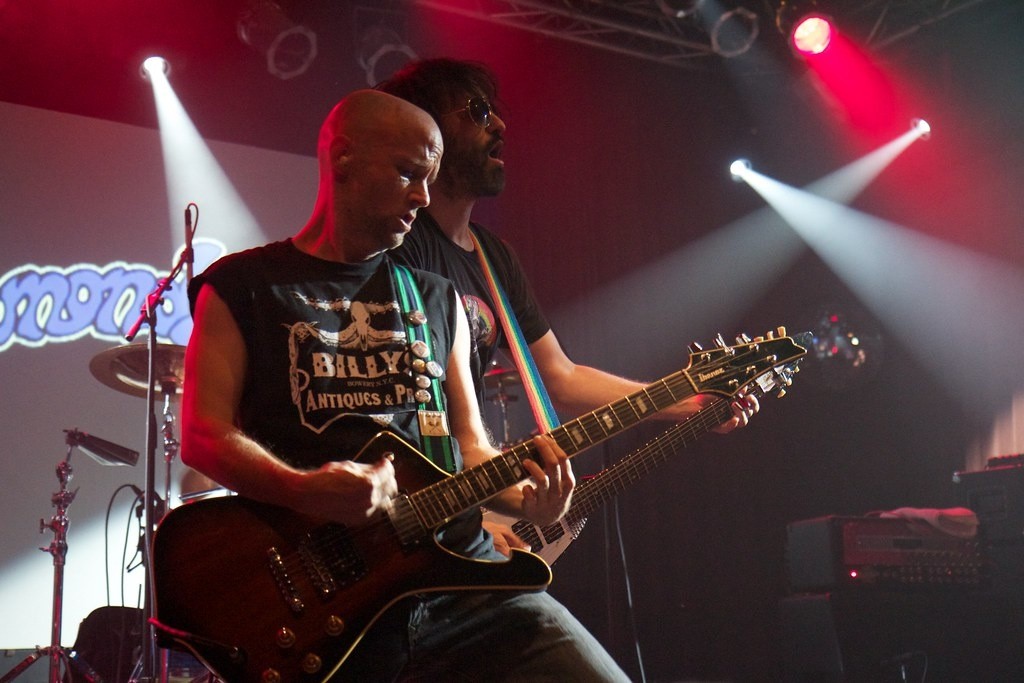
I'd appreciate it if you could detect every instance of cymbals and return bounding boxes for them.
[481,366,531,408]
[89,341,188,400]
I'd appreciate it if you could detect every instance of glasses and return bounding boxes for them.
[436,94,499,129]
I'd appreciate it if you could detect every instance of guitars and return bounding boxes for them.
[150,324,812,682]
[486,356,804,562]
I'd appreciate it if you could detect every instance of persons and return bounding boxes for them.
[179,89,630,682]
[374,56,760,433]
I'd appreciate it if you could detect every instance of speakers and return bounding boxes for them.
[784,541,1024,683]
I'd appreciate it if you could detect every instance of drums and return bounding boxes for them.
[65,606,151,682]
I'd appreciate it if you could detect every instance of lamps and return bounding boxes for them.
[697,1,762,58]
[778,0,835,57]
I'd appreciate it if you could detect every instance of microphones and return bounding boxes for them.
[185,209,194,287]
[64,429,139,467]
[880,651,924,671]
[131,485,146,505]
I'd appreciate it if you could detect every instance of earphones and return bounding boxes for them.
[340,155,351,167]
